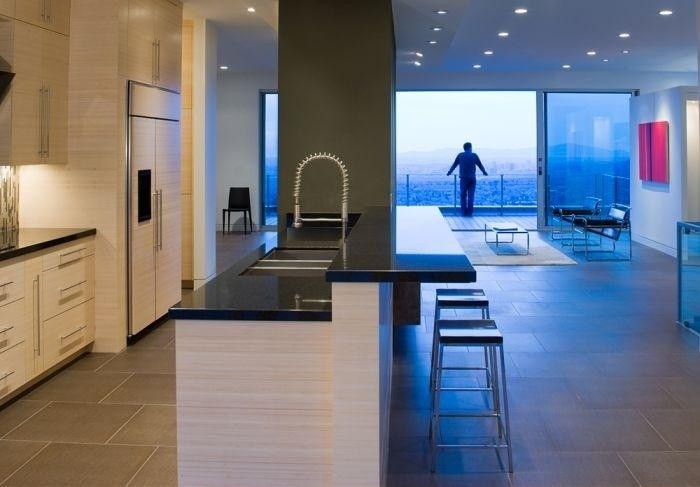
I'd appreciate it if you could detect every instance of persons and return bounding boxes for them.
[446,142,488,216]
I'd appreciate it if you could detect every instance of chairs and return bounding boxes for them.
[220,185,253,235]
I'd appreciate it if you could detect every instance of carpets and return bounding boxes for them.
[453,224,577,266]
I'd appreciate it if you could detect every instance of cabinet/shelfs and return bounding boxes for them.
[0,0,14,18]
[1,18,68,167]
[40,222,98,383]
[0,242,40,409]
[15,0,70,37]
[69,0,186,93]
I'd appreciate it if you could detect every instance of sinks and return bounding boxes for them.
[258,247,340,263]
[248,260,333,270]
[238,268,326,278]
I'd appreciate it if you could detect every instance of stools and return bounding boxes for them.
[551,195,604,248]
[426,317,513,479]
[568,202,633,263]
[430,287,501,420]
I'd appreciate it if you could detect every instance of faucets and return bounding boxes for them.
[292,151,349,242]
